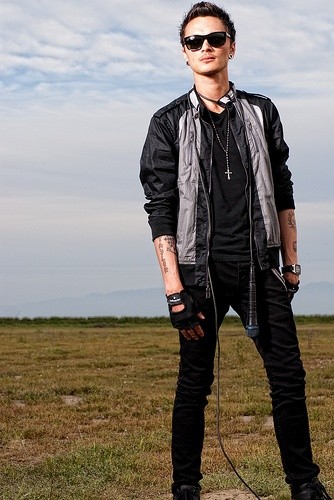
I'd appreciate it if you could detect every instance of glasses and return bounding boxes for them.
[182,32,235,51]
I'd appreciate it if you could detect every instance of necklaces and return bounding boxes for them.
[206,107,232,179]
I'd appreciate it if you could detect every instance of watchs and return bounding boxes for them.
[281,263,301,275]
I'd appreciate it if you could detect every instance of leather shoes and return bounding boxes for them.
[173,485,201,500]
[290,478,332,500]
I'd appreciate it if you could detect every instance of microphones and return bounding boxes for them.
[246,262,259,337]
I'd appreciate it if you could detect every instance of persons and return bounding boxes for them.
[140,1,334,500]
